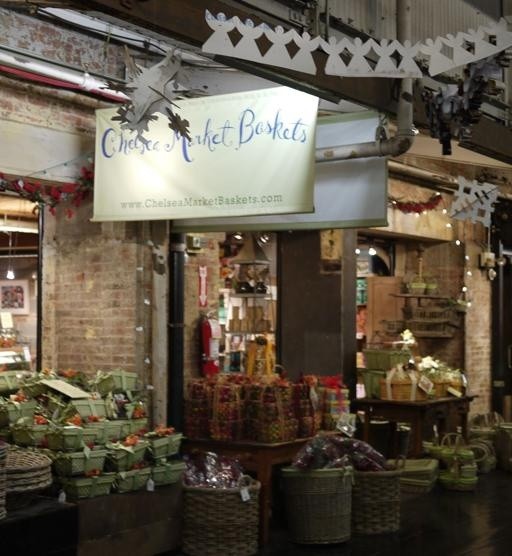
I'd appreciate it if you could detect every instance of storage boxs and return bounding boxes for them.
[60,476,115,500]
[112,418,148,440]
[53,450,107,476]
[0,371,32,391]
[9,423,48,446]
[102,441,152,471]
[318,387,349,400]
[320,400,350,414]
[363,349,411,370]
[94,372,139,398]
[45,429,97,450]
[324,413,356,431]
[84,421,124,444]
[380,379,429,401]
[68,399,107,422]
[148,433,182,458]
[104,467,152,494]
[0,400,37,426]
[149,460,188,485]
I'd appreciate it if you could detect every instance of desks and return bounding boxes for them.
[184,432,347,544]
[359,396,477,462]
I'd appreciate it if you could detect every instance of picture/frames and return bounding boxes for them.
[0,278,31,316]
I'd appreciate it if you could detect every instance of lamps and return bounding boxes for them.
[6,232,15,280]
